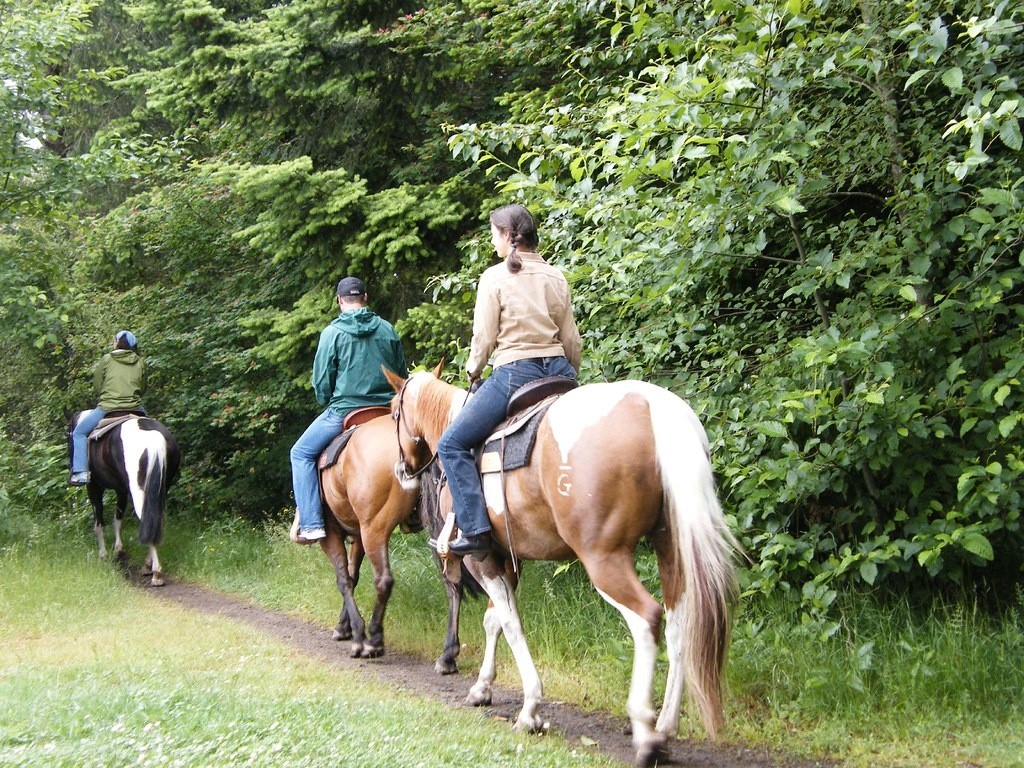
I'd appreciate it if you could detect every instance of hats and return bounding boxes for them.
[336,276,366,304]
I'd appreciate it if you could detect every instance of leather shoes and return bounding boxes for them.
[428,532,492,554]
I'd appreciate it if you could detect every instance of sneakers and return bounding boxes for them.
[71,470,91,483]
[296,527,326,540]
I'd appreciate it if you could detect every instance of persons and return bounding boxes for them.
[437,204,582,561]
[70,331,147,483]
[290,277,409,540]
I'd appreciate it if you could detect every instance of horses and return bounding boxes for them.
[62,405,181,587]
[381,356,752,768]
[315,412,491,658]
